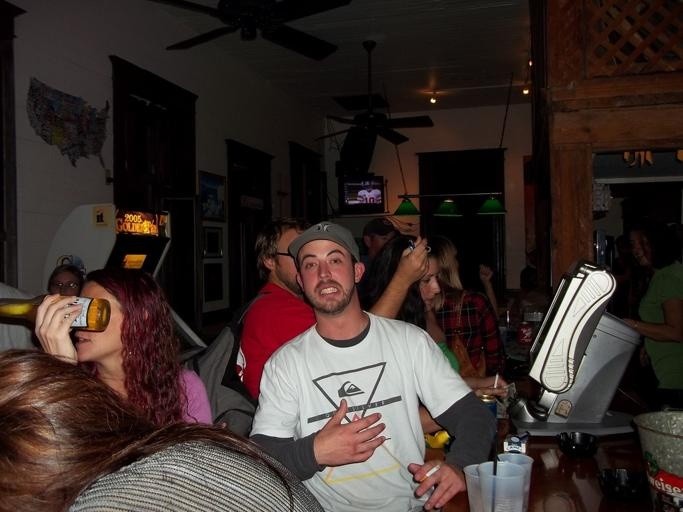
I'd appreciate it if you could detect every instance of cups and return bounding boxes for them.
[631,410,683,512]
[464,453,534,511]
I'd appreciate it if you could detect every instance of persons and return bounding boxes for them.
[614,215,682,409]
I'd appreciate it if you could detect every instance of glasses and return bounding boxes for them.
[49,281,80,291]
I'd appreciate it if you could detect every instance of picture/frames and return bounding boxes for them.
[197,169,227,224]
[201,226,223,258]
[203,262,223,303]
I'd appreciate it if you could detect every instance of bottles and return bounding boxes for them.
[1,293,112,332]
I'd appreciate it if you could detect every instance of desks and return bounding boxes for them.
[439,370,682,511]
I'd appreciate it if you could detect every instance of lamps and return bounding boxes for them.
[379,70,512,217]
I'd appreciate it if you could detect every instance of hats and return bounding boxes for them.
[362,217,400,237]
[288,220,361,265]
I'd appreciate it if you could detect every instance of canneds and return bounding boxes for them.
[518,321,533,345]
[480,395,497,418]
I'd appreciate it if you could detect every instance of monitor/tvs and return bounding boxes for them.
[337,175,386,214]
[528,258,618,394]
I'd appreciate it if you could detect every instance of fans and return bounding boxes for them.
[312,38,434,144]
[156,0,352,62]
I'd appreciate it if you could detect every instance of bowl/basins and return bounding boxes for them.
[599,468,644,500]
[557,431,598,461]
